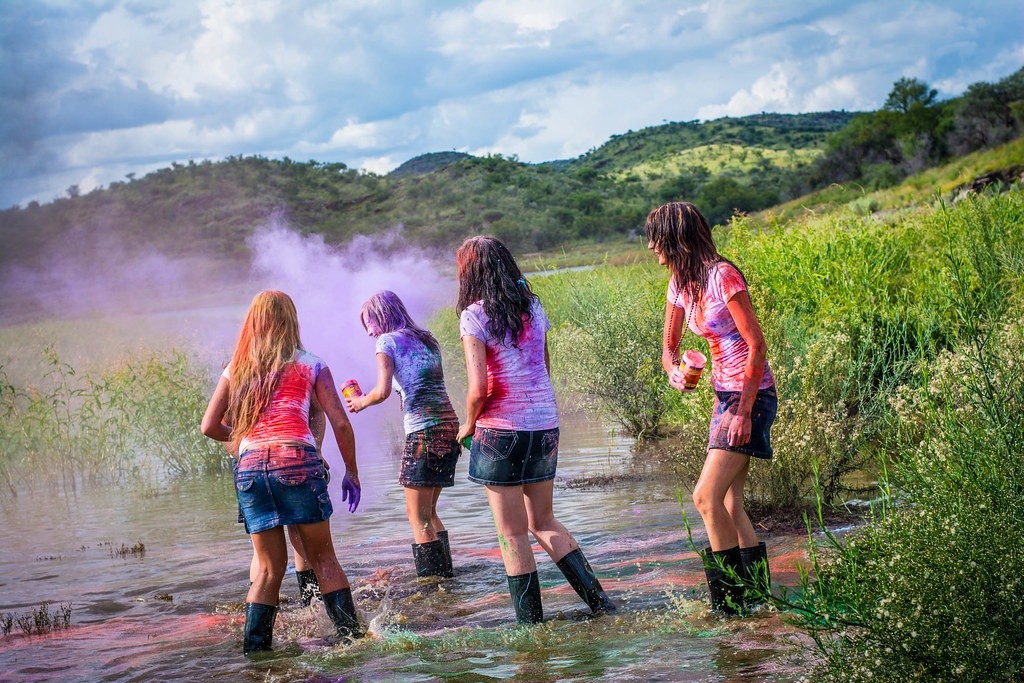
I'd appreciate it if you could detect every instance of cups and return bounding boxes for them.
[341,380,363,404]
[679,350,707,392]
[461,435,473,451]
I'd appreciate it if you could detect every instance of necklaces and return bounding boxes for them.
[667,263,713,366]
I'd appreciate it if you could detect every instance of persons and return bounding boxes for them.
[203,289,362,653]
[456,235,617,626]
[346,291,460,580]
[647,202,779,617]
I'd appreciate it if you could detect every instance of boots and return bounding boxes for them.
[321,588,360,638]
[739,541,771,594]
[506,569,543,624]
[411,538,448,578]
[436,530,452,572]
[251,582,280,609]
[244,603,278,657]
[556,547,615,613]
[295,569,322,610]
[701,544,747,617]
